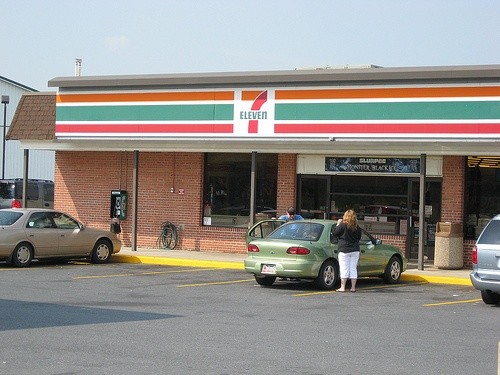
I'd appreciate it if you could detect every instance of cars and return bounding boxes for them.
[0,208,122,267]
[244,219,406,289]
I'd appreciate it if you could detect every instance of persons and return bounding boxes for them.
[334,209,361,292]
[272,206,304,236]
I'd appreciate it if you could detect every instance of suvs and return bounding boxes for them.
[470,214,500,305]
[0,178,55,208]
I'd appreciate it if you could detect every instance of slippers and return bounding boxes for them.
[351,291,356,292]
[336,289,345,292]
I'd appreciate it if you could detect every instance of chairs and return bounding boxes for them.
[33,218,45,226]
